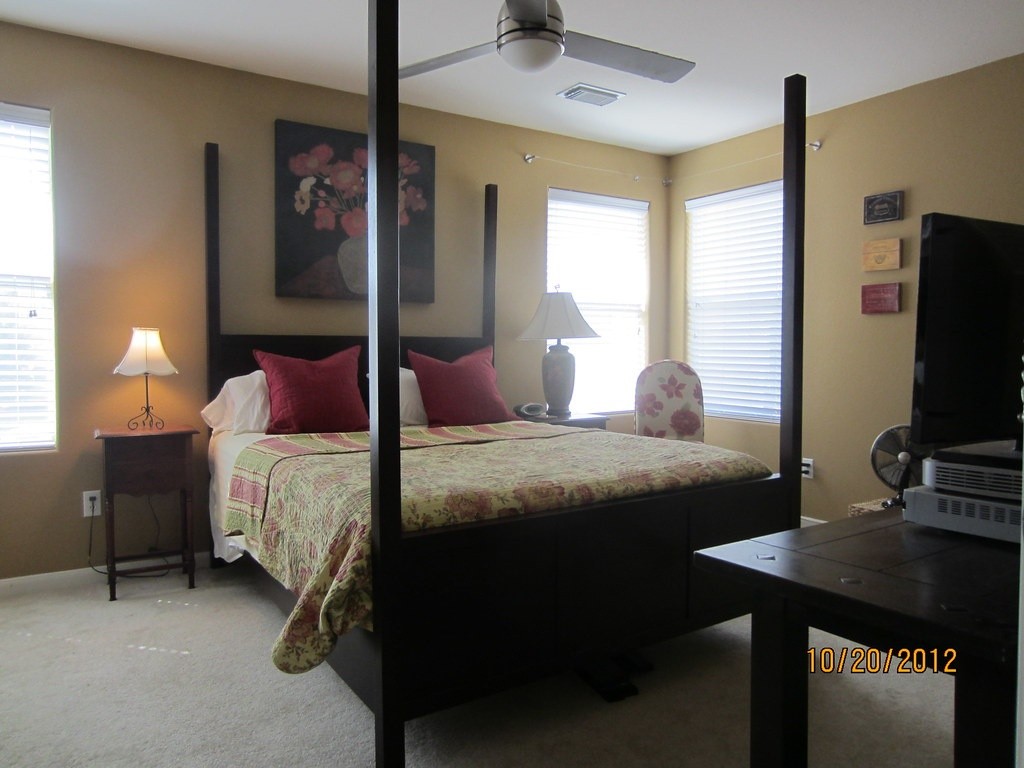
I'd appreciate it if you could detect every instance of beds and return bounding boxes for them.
[203,73,808,768]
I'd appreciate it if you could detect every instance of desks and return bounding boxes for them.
[690,506,1021,767]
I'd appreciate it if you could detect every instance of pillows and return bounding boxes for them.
[200,370,271,433]
[399,367,428,426]
[252,344,371,435]
[407,345,522,426]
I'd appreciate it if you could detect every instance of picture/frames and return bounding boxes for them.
[273,118,435,303]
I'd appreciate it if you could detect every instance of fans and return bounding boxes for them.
[870,423,914,507]
[400,0,695,84]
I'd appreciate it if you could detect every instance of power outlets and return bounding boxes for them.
[82,489,101,517]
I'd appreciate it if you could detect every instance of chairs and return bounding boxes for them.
[633,360,704,442]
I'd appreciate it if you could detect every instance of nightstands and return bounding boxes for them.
[525,413,615,430]
[94,423,201,601]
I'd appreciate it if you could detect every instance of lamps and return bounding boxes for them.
[514,282,602,416]
[110,326,181,431]
[496,32,569,71]
